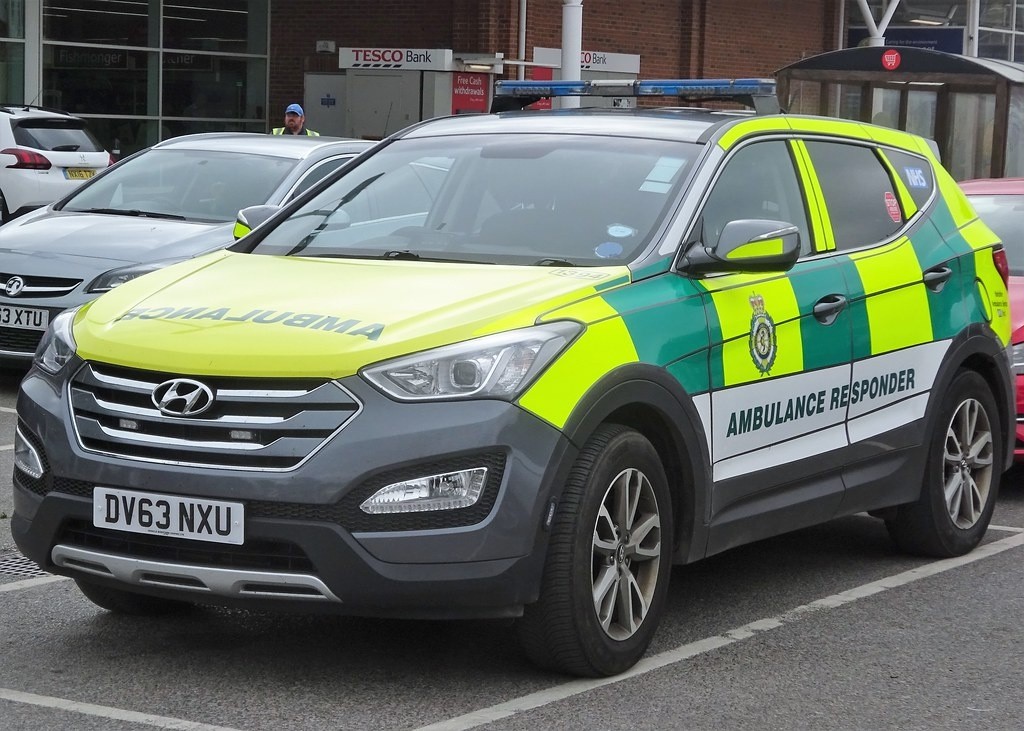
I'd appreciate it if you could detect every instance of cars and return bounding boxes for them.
[1,131,507,390]
[940,178,1022,464]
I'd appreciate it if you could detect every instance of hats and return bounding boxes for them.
[285,104,305,116]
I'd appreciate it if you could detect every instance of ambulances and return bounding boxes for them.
[9,78,1017,679]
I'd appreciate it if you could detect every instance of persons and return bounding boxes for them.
[267,103,320,136]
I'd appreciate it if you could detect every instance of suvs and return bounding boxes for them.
[0,104,125,235]
